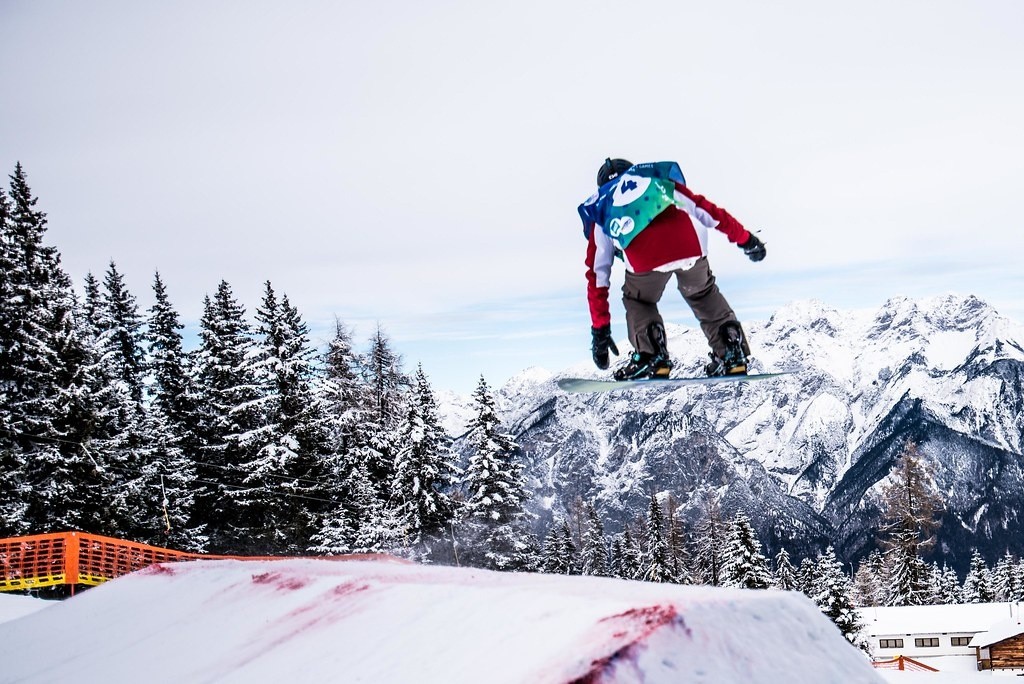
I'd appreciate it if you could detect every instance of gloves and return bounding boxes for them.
[591,325,620,371]
[736,230,766,263]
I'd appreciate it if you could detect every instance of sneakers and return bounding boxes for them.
[704,320,747,374]
[614,321,673,380]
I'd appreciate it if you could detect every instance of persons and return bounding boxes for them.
[578,157,766,381]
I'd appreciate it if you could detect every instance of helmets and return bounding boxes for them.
[597,158,634,186]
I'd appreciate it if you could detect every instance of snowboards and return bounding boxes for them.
[556,370,795,393]
